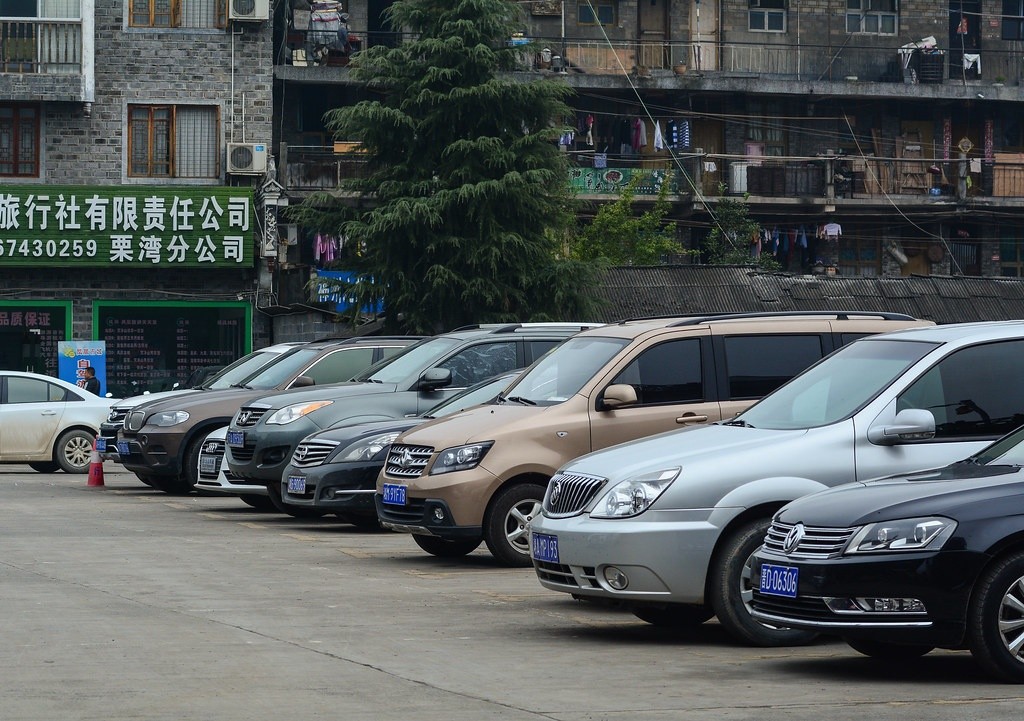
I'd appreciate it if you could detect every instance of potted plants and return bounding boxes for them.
[994,73,1008,88]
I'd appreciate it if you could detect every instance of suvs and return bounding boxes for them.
[96,333,432,498]
[374,310,943,567]
[225,322,608,513]
[528,319,1023,649]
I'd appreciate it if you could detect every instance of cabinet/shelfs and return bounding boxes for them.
[327,40,363,67]
[838,169,867,196]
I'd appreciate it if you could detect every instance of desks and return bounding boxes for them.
[746,165,825,195]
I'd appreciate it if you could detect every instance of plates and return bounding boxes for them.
[603,170,623,184]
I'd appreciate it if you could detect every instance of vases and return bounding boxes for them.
[673,65,687,74]
[812,266,835,276]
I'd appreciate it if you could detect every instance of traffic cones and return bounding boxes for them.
[82,439,105,491]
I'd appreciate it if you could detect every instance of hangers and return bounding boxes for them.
[558,108,689,126]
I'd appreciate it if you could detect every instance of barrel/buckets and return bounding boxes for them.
[552,58,562,66]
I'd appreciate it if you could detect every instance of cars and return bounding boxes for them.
[0,370,124,474]
[281,363,557,533]
[746,423,1023,684]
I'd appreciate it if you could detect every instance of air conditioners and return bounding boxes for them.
[276,223,298,246]
[229,0,269,22]
[227,142,267,175]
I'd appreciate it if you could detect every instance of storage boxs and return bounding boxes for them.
[847,158,865,172]
[312,12,341,35]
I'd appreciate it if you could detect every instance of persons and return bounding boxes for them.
[83,367,100,396]
